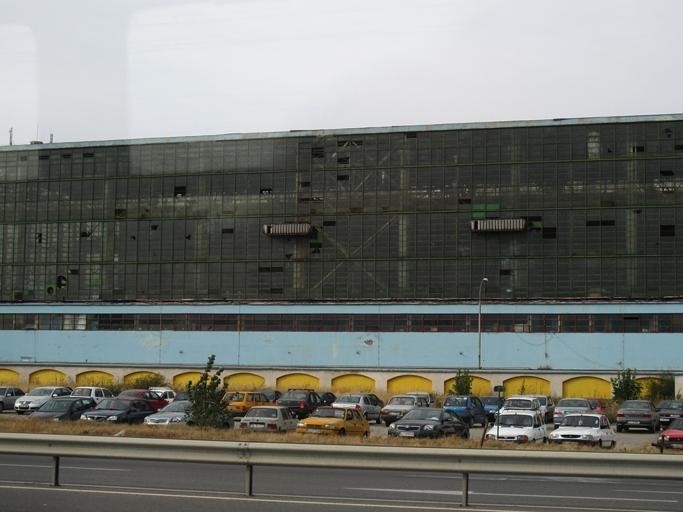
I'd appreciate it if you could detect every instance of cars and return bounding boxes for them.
[616,399,661,433]
[658,418,682,452]
[655,401,683,426]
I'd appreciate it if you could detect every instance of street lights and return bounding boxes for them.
[477,276,489,367]
